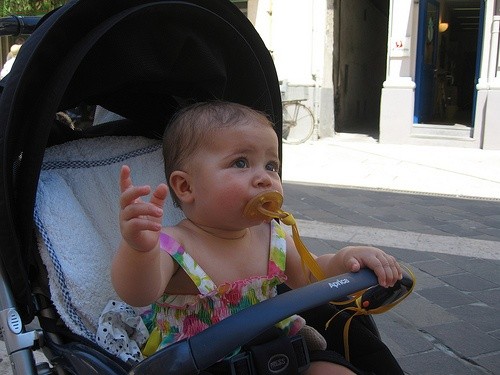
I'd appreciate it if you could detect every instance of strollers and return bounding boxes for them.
[0,1,416,375]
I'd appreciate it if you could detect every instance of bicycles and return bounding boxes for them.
[282,94,316,147]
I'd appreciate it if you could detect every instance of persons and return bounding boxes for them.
[113,102,403,375]
